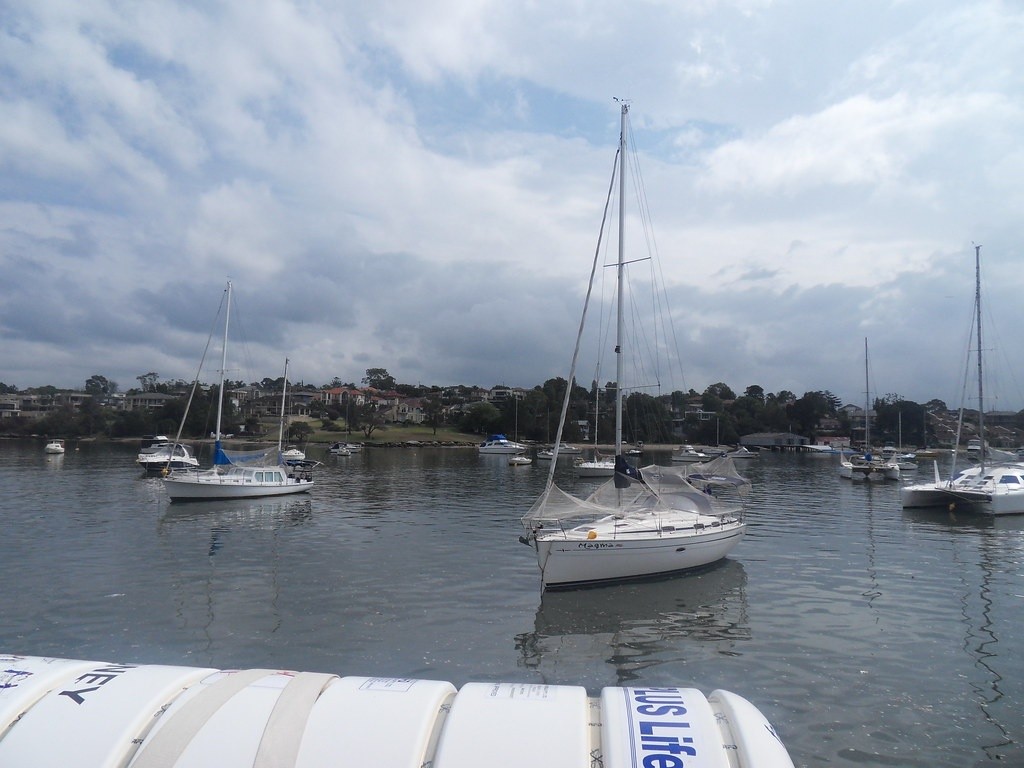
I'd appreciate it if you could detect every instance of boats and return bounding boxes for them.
[898,459,957,509]
[669,439,712,462]
[327,403,363,456]
[625,448,644,456]
[475,434,528,454]
[44,439,65,455]
[881,446,917,460]
[726,446,760,459]
[550,440,583,454]
[966,439,990,462]
[507,450,533,465]
[281,445,305,461]
[134,435,200,471]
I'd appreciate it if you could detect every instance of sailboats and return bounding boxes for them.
[517,96,752,594]
[886,394,920,471]
[932,240,1024,517]
[153,277,324,499]
[535,407,558,459]
[572,361,630,478]
[839,336,901,482]
